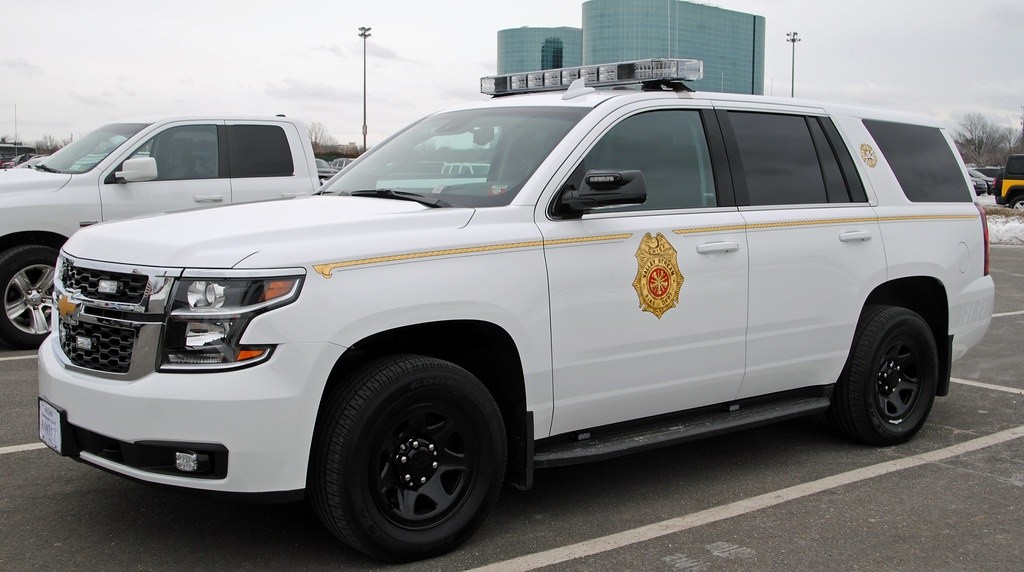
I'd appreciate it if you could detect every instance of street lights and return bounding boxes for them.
[786,32,801,98]
[359,28,373,156]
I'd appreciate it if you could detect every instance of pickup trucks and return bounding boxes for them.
[0,111,487,351]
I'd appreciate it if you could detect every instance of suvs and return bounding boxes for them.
[994,152,1024,209]
[36,58,997,564]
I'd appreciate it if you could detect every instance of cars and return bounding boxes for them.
[315,156,491,184]
[968,164,1003,194]
[0,152,45,171]
